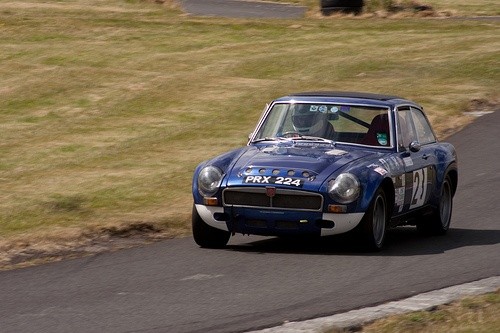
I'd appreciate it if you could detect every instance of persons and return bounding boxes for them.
[285,104,339,141]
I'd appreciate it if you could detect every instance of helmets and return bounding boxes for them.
[291,105,328,136]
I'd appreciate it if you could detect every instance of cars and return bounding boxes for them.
[191,90,459,248]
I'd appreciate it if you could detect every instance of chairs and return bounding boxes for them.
[362,114,390,147]
